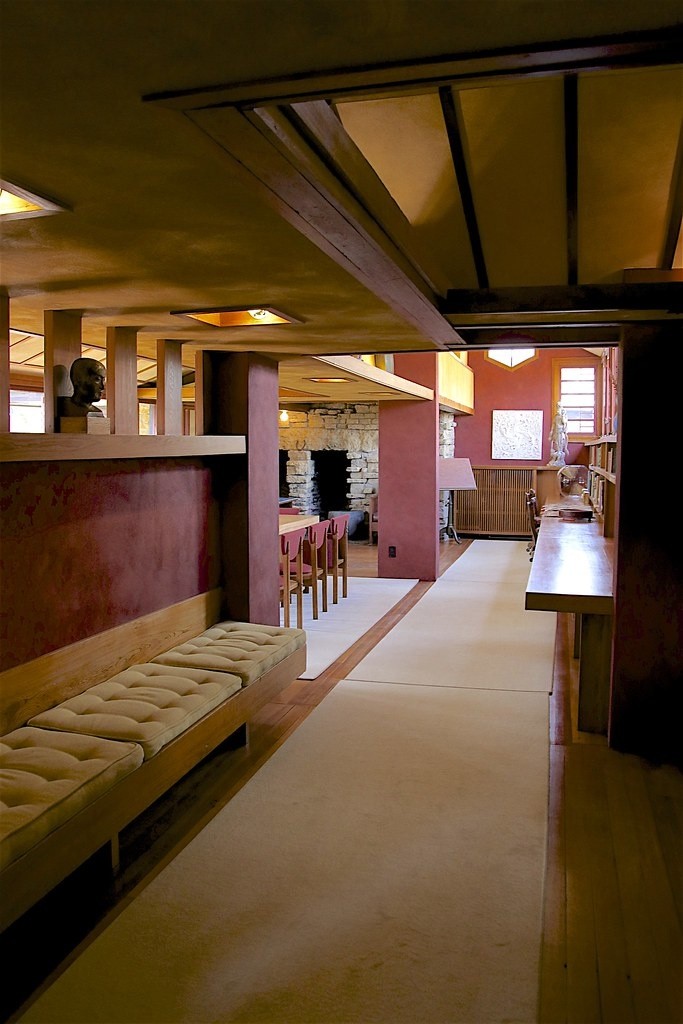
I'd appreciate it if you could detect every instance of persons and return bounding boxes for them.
[57,358,105,416]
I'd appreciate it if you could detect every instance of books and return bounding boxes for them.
[587,444,616,514]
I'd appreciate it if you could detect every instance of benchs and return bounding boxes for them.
[0,619,308,933]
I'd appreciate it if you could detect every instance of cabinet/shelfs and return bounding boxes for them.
[525,435,616,732]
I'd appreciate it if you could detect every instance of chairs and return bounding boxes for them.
[278,507,349,629]
[525,490,541,562]
[368,493,378,545]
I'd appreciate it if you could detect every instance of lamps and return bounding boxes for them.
[439,458,477,544]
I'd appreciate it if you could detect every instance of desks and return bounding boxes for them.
[327,511,365,541]
[279,497,298,508]
[279,514,319,536]
